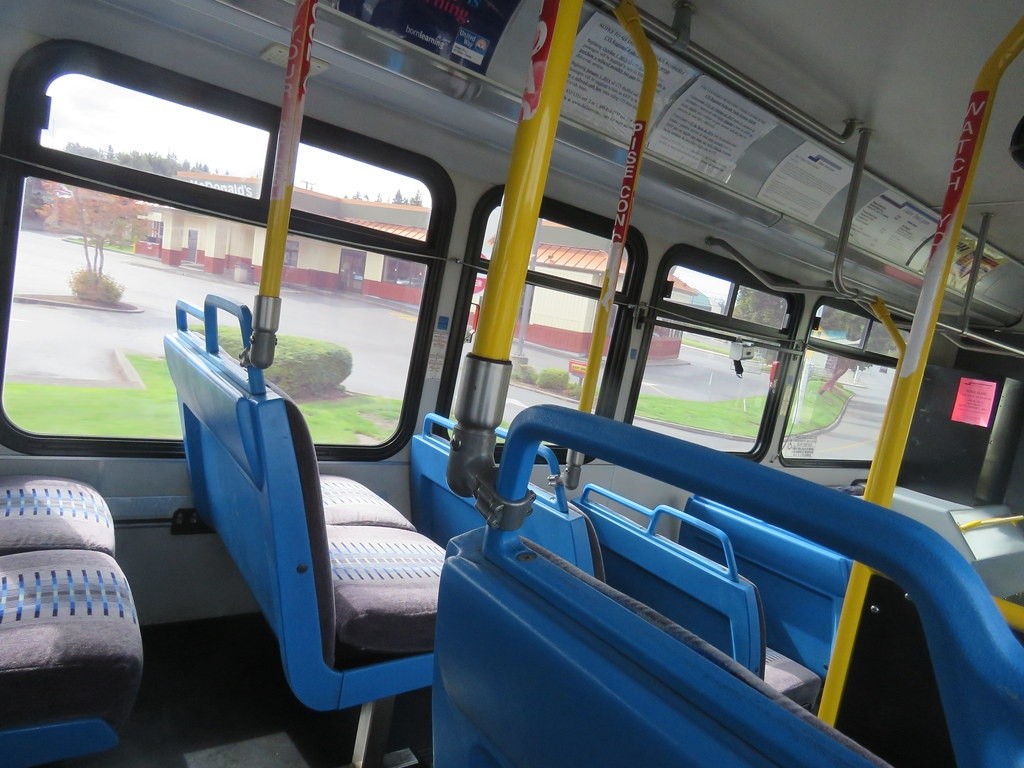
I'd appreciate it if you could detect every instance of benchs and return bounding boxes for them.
[0,472,144,768]
[164,293,447,768]
[412,403,1024,768]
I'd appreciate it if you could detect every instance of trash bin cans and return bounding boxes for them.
[235,264,248,283]
